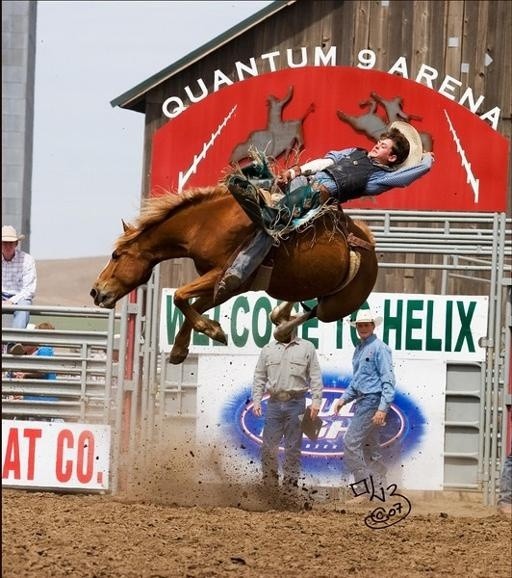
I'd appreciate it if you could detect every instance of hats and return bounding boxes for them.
[2,225,26,242]
[349,309,383,329]
[387,121,423,169]
[268,302,305,324]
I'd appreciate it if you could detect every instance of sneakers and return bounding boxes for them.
[7,344,25,355]
[218,273,241,297]
[344,493,370,508]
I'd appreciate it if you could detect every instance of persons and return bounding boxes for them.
[212,116,438,307]
[332,307,397,507]
[250,305,326,510]
[2,223,38,356]
[6,321,59,424]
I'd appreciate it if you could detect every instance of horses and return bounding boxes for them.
[87,177,382,366]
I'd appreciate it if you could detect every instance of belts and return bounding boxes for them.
[271,391,305,402]
